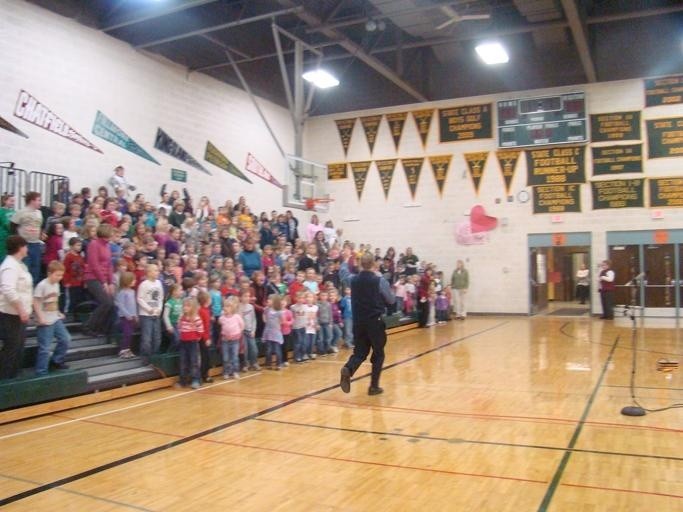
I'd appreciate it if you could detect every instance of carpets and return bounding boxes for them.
[547,305,590,317]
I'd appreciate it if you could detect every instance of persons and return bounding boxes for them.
[339,254,395,396]
[450,260,468,320]
[598,261,615,319]
[289,214,452,363]
[530,276,537,290]
[0,165,300,390]
[576,264,589,305]
[626,270,650,304]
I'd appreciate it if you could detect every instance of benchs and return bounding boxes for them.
[112,329,180,376]
[0,324,55,373]
[380,308,416,328]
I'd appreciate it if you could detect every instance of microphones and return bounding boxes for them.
[616,305,644,310]
[631,268,650,282]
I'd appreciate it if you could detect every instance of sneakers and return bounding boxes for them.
[173,342,383,395]
